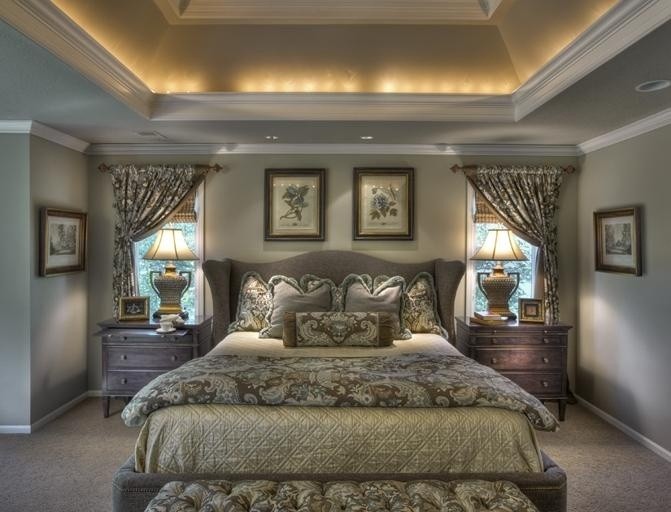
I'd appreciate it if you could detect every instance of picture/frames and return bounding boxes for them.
[37,205,88,276]
[262,165,328,245]
[592,204,641,279]
[352,166,415,241]
[117,295,149,323]
[517,297,545,324]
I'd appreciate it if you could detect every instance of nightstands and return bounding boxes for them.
[90,316,214,417]
[452,314,573,422]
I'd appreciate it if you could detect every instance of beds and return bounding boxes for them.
[107,247,580,512]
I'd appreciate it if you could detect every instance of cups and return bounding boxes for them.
[159,315,186,328]
[161,322,174,331]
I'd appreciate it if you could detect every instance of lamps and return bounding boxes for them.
[141,226,200,317]
[469,228,529,314]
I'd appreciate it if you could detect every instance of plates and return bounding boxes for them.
[155,328,177,333]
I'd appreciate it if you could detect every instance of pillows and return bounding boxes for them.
[297,272,371,305]
[225,269,298,336]
[370,269,450,340]
[258,271,335,340]
[339,272,413,341]
[281,310,393,349]
